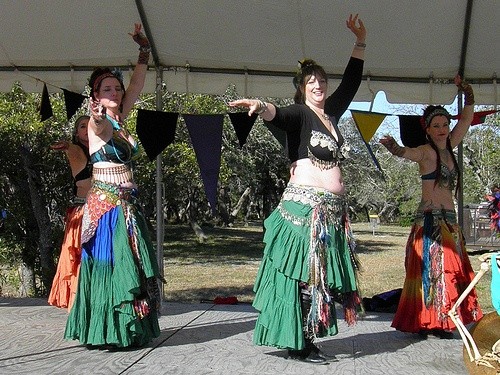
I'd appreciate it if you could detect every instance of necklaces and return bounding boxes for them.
[310,103,330,121]
[106,111,127,135]
[437,143,451,165]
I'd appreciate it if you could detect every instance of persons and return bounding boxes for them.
[379,74,483,340]
[48,22,167,348]
[228,13,368,365]
[49,115,90,308]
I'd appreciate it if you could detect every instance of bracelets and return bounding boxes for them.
[353,40,368,49]
[255,98,268,115]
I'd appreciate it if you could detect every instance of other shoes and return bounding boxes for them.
[307,344,338,361]
[288,350,328,365]
